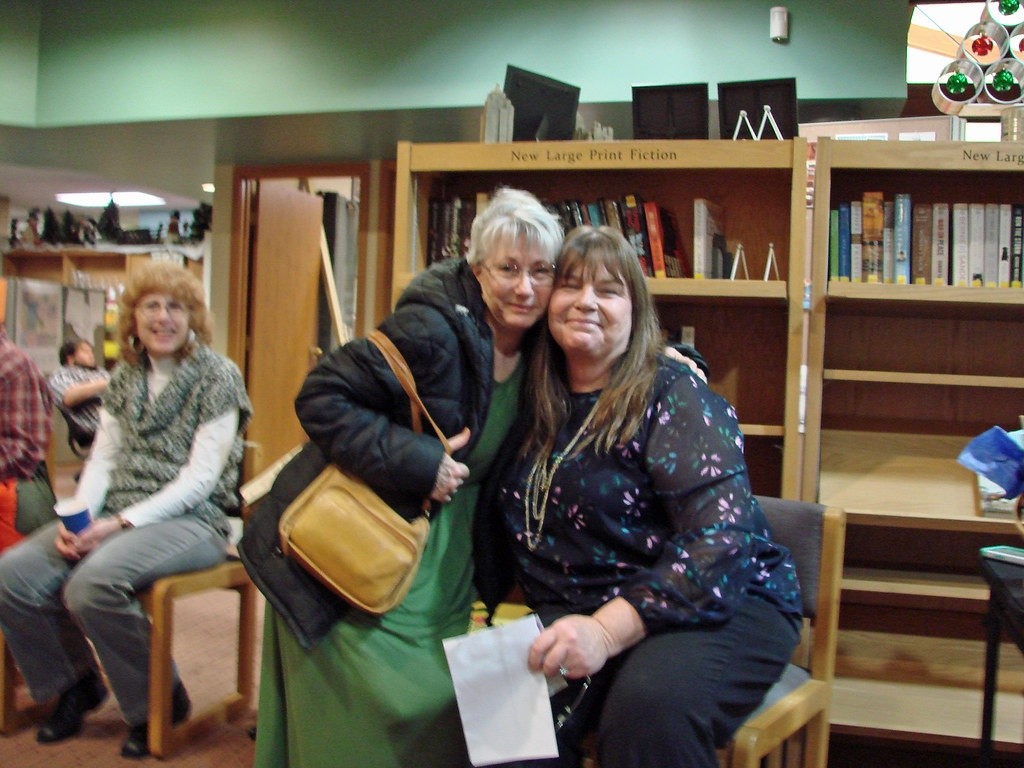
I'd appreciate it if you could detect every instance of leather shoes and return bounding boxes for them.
[35,678,105,743]
[124,685,190,755]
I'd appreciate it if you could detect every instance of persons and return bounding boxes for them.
[239,191,710,768]
[500,228,804,768]
[0,263,254,758]
[48,339,110,434]
[0,325,54,476]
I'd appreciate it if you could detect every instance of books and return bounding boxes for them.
[978,474,1015,517]
[427,193,491,265]
[830,192,1024,288]
[661,325,696,349]
[543,195,723,279]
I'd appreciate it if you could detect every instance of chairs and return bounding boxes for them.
[0,441,262,756]
[726,495,847,768]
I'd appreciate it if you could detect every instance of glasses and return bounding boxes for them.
[479,260,558,287]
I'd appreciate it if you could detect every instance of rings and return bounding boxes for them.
[559,664,569,676]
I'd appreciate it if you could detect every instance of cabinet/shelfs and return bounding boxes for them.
[802,136,1024,611]
[393,137,810,497]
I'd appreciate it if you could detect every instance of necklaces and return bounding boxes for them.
[524,399,601,551]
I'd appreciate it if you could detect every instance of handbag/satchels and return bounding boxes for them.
[281,465,420,614]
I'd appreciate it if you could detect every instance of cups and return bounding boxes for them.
[53,496,90,534]
